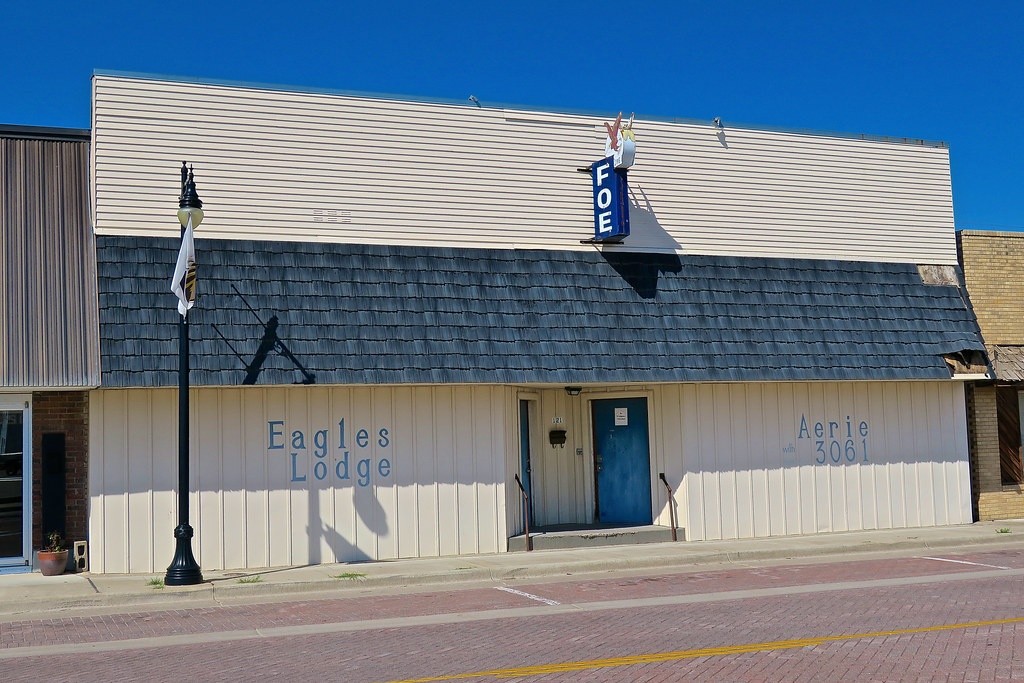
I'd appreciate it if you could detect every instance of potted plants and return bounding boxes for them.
[36,529,69,577]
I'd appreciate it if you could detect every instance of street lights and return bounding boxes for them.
[164,159,207,586]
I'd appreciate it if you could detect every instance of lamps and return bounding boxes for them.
[564,386,582,395]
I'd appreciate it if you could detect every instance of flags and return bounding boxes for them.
[171,215,196,323]
